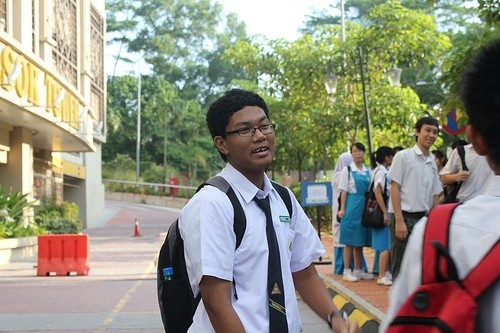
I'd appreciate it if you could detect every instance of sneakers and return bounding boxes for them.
[377,271,393,285]
[353,268,374,280]
[342,272,358,281]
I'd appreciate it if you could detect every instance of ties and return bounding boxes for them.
[252,195,289,333]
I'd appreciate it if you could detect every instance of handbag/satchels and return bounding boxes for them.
[362,189,389,229]
[336,191,344,222]
[441,145,468,204]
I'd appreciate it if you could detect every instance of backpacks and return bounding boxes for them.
[384,202,500,333]
[156,176,292,333]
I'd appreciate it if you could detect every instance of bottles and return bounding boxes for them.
[161,267,177,280]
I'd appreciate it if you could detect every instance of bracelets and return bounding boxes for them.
[327,311,343,329]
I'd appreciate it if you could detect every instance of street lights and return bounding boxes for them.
[322,45,403,170]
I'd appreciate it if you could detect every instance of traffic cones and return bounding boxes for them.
[131,217,143,237]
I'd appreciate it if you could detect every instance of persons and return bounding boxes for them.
[179,88,361,333]
[380,39,500,333]
[332,118,500,285]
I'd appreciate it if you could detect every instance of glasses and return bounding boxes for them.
[221,124,276,137]
